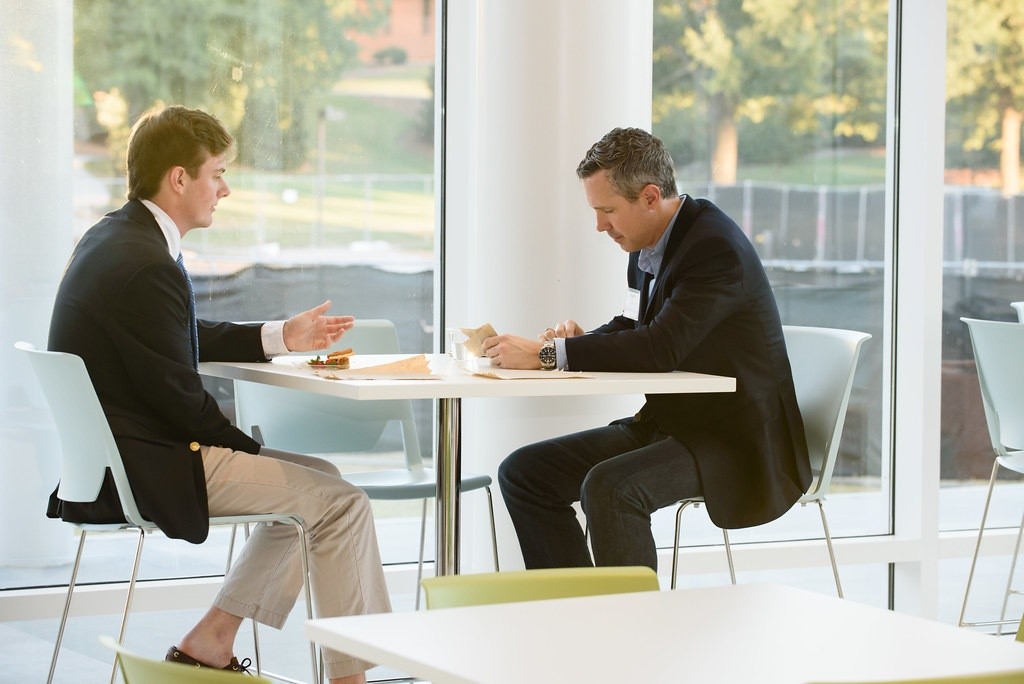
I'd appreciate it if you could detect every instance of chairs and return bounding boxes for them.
[95,633,273,684]
[225,318,499,684]
[584,324,872,598]
[13,340,320,684]
[421,565,659,610]
[958,317,1023,631]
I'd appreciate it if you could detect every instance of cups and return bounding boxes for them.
[449,331,468,360]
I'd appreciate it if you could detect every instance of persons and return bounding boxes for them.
[45,106,393,683]
[481,125,815,586]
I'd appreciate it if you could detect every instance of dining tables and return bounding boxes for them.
[197,354,735,575]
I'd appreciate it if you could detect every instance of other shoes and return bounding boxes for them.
[164,641,252,675]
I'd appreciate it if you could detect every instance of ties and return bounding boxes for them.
[178,251,199,373]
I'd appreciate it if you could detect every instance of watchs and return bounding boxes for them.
[538,340,559,371]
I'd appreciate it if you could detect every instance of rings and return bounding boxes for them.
[544,328,555,333]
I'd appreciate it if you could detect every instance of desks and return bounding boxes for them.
[304,585,1023,684]
